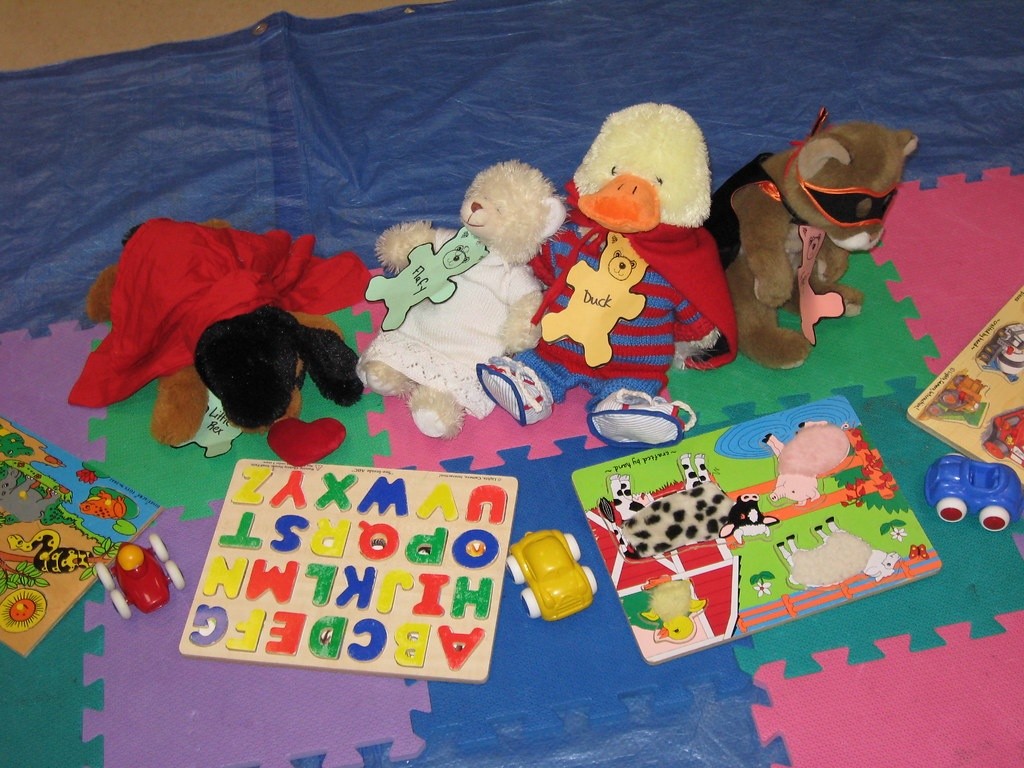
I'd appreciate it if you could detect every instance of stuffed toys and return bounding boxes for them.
[703,122,916,368]
[476,104,737,448]
[356,159,566,439]
[66,220,372,444]
